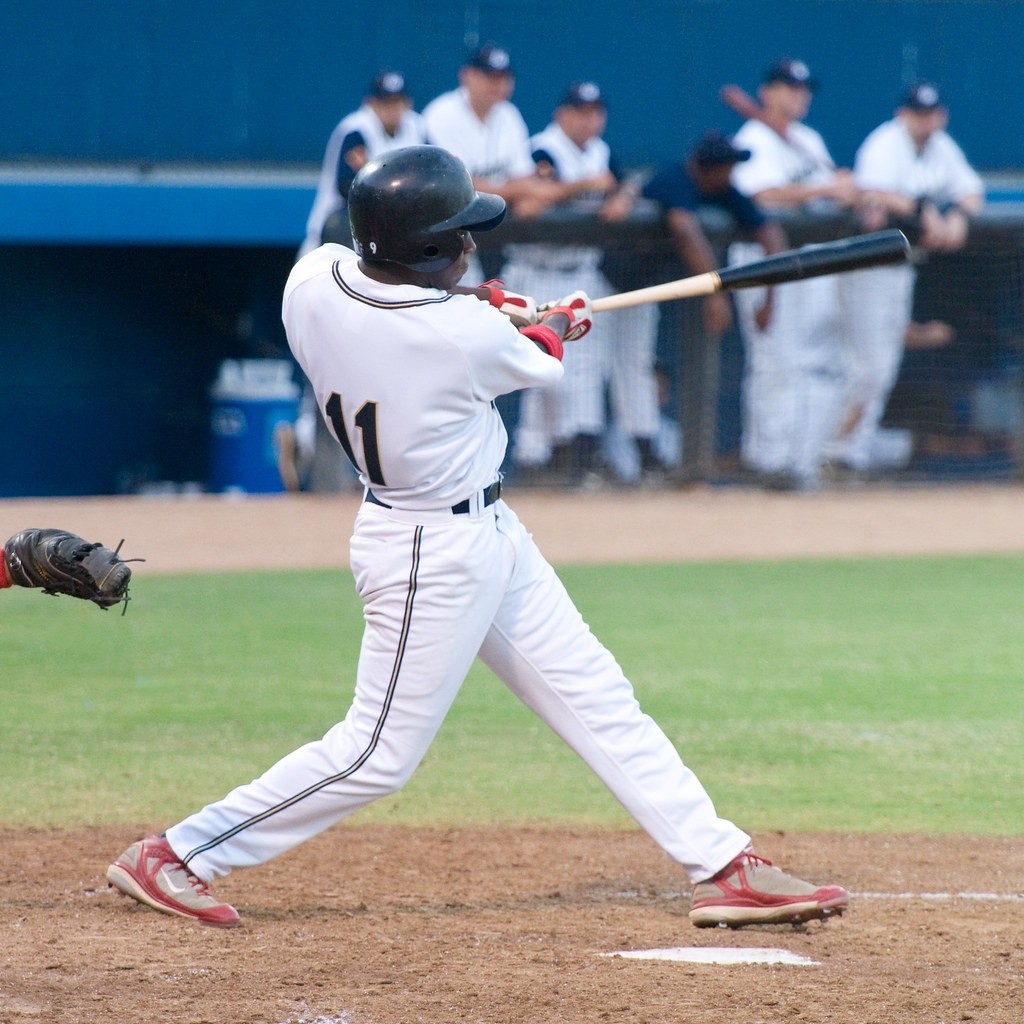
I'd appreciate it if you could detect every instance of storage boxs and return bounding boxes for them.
[207,357,309,494]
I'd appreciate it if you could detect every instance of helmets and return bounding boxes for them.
[348,146,505,273]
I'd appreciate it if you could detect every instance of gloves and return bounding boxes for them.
[520,289,594,363]
[477,277,538,331]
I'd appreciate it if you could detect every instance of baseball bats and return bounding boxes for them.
[535,225,912,321]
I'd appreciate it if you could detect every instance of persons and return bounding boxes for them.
[0,528,146,616]
[293,49,790,483]
[728,57,986,488]
[105,145,849,933]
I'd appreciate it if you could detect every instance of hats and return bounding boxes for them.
[370,74,413,100]
[761,59,821,95]
[901,85,946,114]
[464,48,515,78]
[690,136,750,164]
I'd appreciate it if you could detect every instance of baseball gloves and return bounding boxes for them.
[3,527,147,617]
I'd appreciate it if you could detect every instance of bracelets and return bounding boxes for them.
[956,205,972,219]
[913,195,927,218]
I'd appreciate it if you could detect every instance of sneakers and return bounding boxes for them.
[106,834,241,928]
[688,848,851,929]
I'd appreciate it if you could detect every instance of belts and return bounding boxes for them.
[367,473,505,514]
